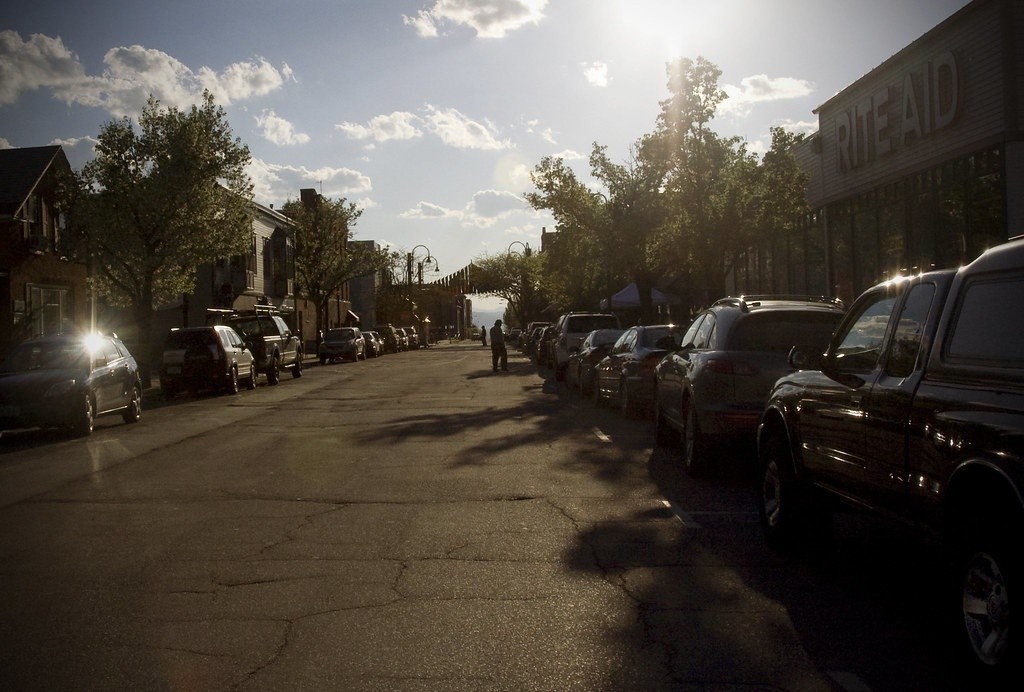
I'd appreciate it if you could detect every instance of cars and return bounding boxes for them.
[396,328,409,349]
[566,328,631,395]
[508,322,557,364]
[369,331,386,354]
[362,332,379,358]
[159,326,257,398]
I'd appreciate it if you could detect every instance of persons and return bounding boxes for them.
[481,326,487,346]
[490,319,509,372]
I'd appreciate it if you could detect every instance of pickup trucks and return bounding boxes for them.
[218,309,303,384]
[754,233,1024,680]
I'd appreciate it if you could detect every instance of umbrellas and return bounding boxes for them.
[601,281,668,321]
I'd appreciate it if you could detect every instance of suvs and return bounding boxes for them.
[546,311,624,381]
[653,294,845,472]
[319,327,366,365]
[594,325,688,419]
[401,326,420,349]
[0,330,143,436]
[369,323,403,353]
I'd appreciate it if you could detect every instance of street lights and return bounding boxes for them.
[506,242,531,319]
[407,245,431,324]
[418,256,440,325]
